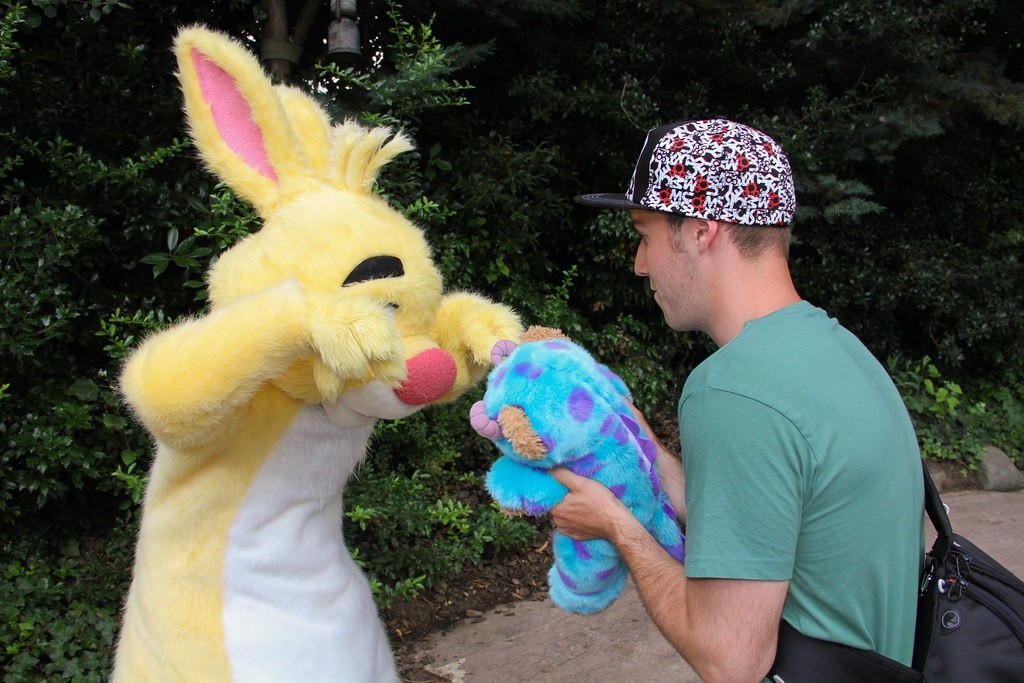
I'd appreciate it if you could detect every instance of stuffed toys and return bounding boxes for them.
[470,325,686,616]
[110,23,525,683]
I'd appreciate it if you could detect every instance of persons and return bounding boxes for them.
[548,118,927,683]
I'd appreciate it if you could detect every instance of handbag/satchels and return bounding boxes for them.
[908,529,1024,683]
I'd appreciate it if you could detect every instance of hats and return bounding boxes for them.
[575,117,795,227]
